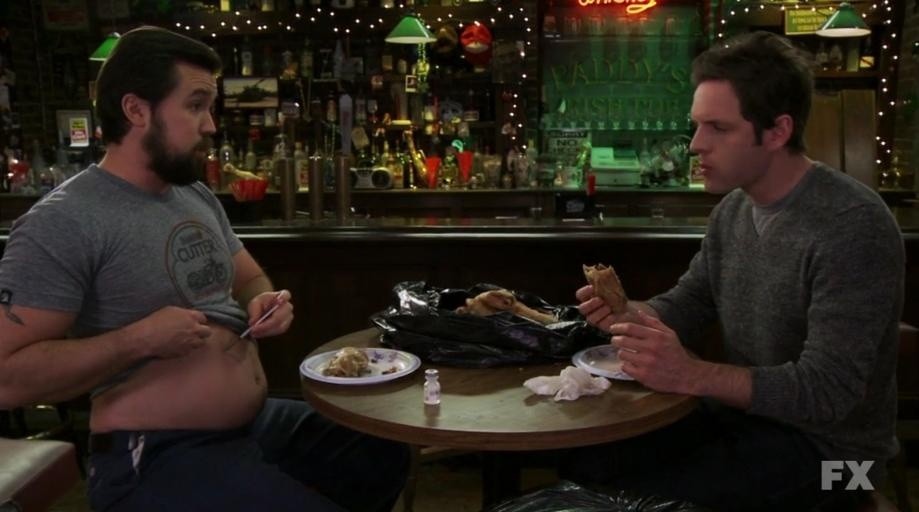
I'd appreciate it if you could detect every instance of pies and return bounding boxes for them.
[581,264,627,318]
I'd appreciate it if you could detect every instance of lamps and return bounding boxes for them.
[89,2,123,63]
[382,2,439,45]
[815,2,872,40]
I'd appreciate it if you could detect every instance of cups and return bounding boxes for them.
[563,166,583,191]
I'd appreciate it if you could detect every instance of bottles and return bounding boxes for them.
[28,137,71,195]
[472,142,495,183]
[639,136,662,184]
[203,30,412,194]
[422,369,442,406]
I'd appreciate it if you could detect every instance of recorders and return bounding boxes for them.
[349,165,394,189]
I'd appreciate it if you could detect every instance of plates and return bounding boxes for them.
[572,344,637,382]
[300,348,421,386]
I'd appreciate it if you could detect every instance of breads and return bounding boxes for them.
[322,346,367,378]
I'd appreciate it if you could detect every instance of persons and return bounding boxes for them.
[1,24,411,510]
[455,28,909,512]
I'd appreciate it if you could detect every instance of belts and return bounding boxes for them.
[87,426,231,453]
